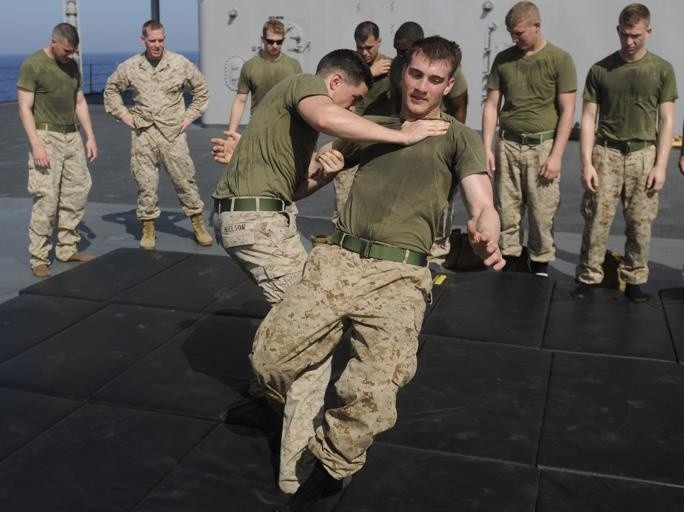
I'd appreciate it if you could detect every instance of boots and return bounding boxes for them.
[310,234,328,248]
[613,256,626,292]
[140,220,155,249]
[602,249,622,269]
[190,214,213,246]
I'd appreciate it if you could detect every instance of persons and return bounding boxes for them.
[482,2,577,274]
[570,3,679,302]
[212,50,450,497]
[679,122,684,174]
[17,23,97,278]
[389,22,467,275]
[227,20,303,141]
[211,36,505,512]
[332,21,391,231]
[104,21,213,252]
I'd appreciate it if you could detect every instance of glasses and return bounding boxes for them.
[264,38,284,45]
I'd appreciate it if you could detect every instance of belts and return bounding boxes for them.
[36,123,80,134]
[330,230,427,266]
[498,128,555,145]
[214,198,283,212]
[596,137,652,154]
